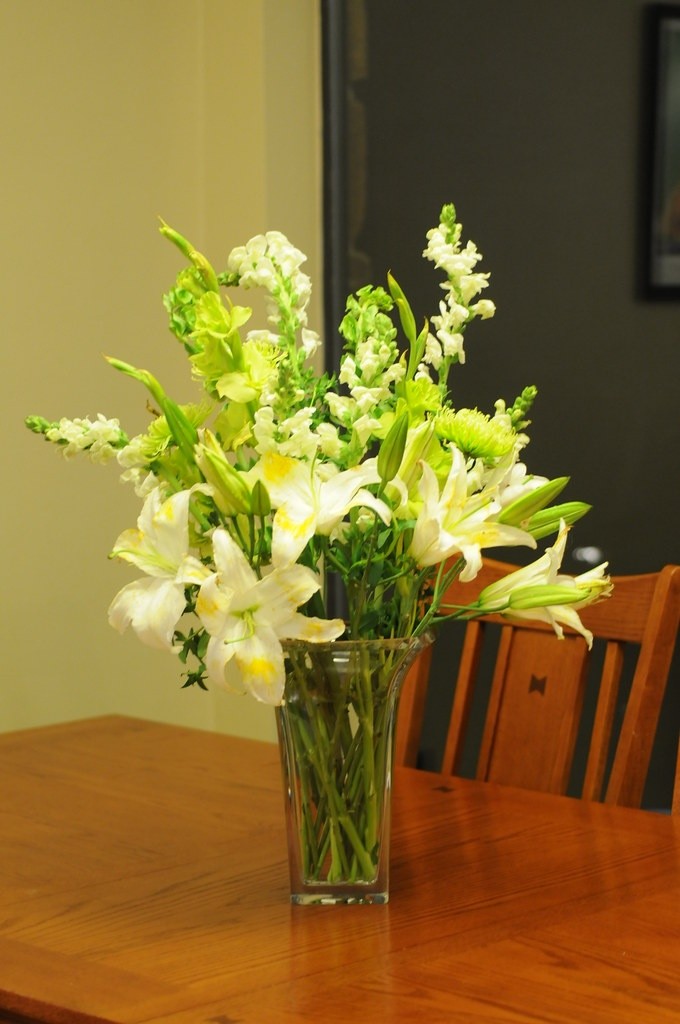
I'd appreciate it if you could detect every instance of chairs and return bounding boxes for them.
[390,552,680,809]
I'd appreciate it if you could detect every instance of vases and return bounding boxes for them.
[272,639,433,908]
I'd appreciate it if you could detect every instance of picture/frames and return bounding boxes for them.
[632,3,680,302]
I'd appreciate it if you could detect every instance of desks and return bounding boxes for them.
[0,713,680,1024]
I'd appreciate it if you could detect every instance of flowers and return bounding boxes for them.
[25,203,616,888]
